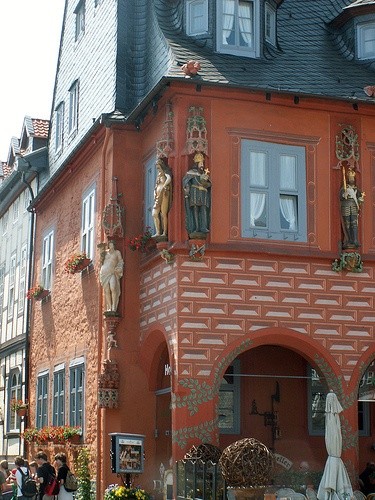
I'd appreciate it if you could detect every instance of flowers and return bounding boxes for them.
[17,424,83,451]
[62,250,89,275]
[26,284,45,305]
[127,225,153,258]
[9,398,23,413]
[103,483,153,500]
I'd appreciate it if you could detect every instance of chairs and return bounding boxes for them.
[275,487,375,500]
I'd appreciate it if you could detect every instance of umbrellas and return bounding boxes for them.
[317,392,354,500]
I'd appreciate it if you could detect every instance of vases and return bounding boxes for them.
[144,238,159,254]
[36,437,49,445]
[32,436,38,442]
[52,436,65,445]
[74,258,91,273]
[17,407,26,416]
[36,289,48,301]
[70,434,81,445]
[226,485,277,500]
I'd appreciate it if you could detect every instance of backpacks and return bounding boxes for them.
[62,466,77,492]
[16,467,37,497]
[40,467,61,496]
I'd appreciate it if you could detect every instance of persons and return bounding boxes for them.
[181,153,211,233]
[36,451,56,500]
[98,240,124,312]
[14,456,31,500]
[0,470,16,500]
[151,161,172,237]
[339,170,366,247]
[29,461,39,482]
[0,460,11,483]
[55,452,74,500]
